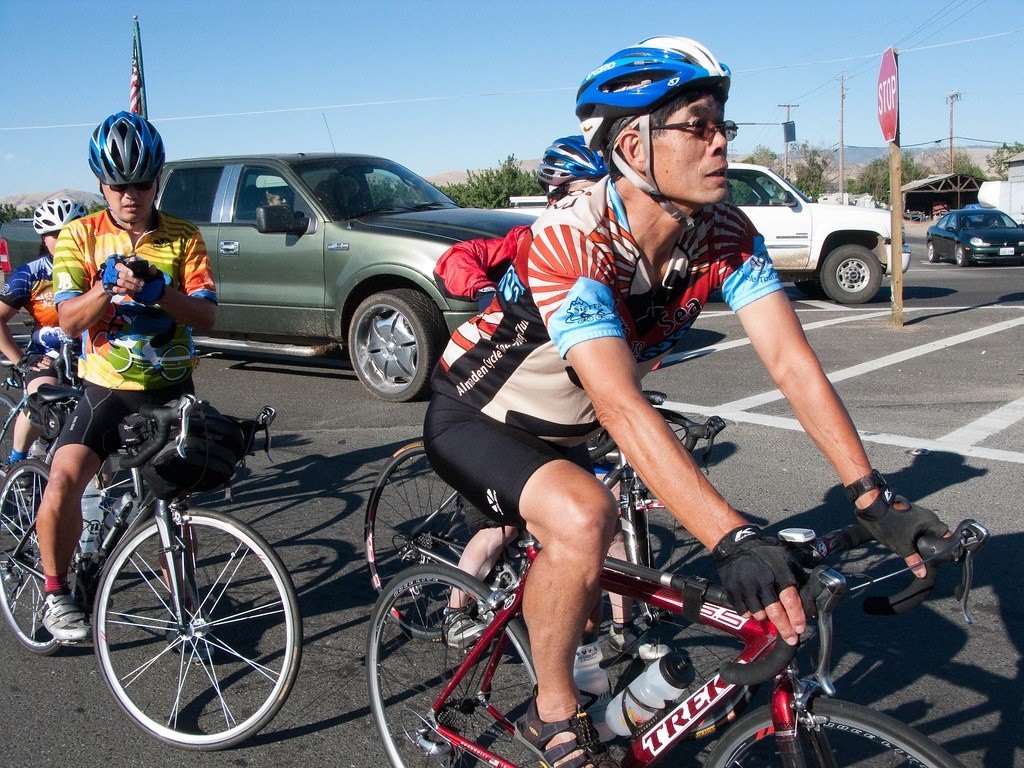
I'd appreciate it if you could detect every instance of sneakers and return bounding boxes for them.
[441,616,487,649]
[607,623,672,662]
[35,591,92,641]
[7,459,33,489]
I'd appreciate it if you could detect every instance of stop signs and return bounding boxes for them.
[875,43,900,144]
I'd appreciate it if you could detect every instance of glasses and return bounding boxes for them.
[634,119,738,142]
[107,182,157,191]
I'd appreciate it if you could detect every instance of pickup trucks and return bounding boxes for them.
[0,151,543,400]
[493,163,913,304]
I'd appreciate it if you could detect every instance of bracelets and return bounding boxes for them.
[843,468,888,507]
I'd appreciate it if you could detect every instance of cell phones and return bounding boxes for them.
[126,260,148,280]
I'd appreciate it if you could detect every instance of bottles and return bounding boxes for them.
[572,630,616,743]
[605,650,695,737]
[93,491,139,553]
[79,474,104,554]
[26,436,49,462]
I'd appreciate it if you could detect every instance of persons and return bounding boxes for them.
[423,34,954,768]
[266,186,295,211]
[438,134,649,653]
[0,197,112,499]
[36,111,220,643]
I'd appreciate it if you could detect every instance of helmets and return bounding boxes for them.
[537,136,611,189]
[88,112,166,186]
[573,36,731,151]
[33,199,87,236]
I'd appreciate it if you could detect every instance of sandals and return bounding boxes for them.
[513,686,617,768]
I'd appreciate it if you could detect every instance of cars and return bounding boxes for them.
[924,204,1024,269]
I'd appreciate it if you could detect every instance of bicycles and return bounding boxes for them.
[367,506,989,768]
[1,325,144,580]
[362,381,726,738]
[0,391,305,751]
